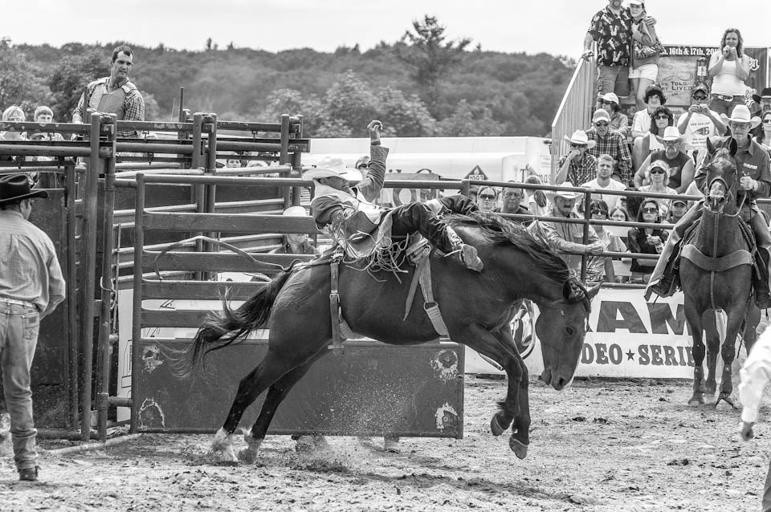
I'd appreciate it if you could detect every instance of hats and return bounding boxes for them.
[301,157,363,182]
[545,182,584,201]
[0,172,48,203]
[645,84,687,144]
[719,107,762,129]
[563,92,619,146]
[752,88,771,102]
[691,85,709,95]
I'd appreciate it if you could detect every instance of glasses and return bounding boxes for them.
[590,169,664,214]
[479,194,495,201]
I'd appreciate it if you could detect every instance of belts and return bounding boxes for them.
[0,297,36,307]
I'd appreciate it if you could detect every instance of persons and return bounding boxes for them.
[583,0,658,99]
[0,106,26,141]
[628,0,663,109]
[71,46,146,142]
[1,173,67,480]
[34,106,65,141]
[738,323,771,512]
[651,105,771,309]
[303,120,491,272]
[226,154,395,205]
[709,30,751,116]
[474,85,771,285]
[251,206,322,281]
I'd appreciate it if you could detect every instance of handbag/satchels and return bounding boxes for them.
[632,39,664,60]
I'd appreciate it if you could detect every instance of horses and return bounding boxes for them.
[154,207,605,467]
[677,136,755,415]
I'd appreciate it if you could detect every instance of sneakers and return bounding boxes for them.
[460,245,484,272]
